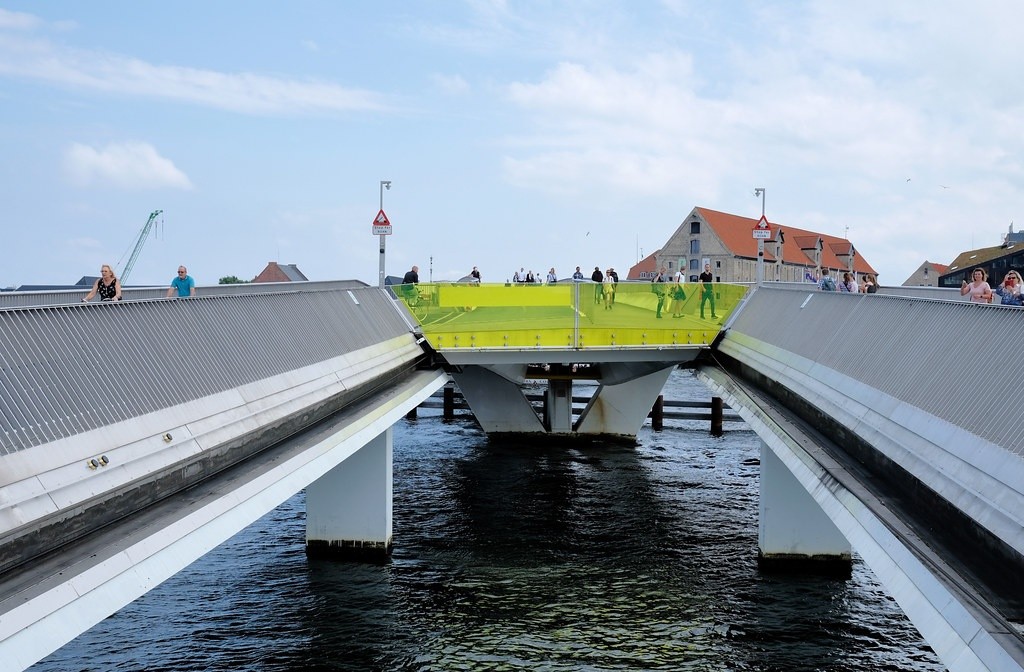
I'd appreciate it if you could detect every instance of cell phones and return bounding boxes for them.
[1005,281,1010,289]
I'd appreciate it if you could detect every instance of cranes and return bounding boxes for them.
[106,210,163,285]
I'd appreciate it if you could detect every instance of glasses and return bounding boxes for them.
[178,271,185,273]
[100,271,108,272]
[1008,277,1017,280]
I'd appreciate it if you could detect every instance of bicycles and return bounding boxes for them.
[402,288,429,322]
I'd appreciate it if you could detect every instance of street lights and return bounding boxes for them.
[753,187,767,284]
[377,180,392,288]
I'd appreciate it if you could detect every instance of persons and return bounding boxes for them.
[699,264,718,320]
[165,265,195,298]
[546,267,557,286]
[836,273,854,293]
[818,269,837,291]
[592,266,603,304]
[609,268,618,304]
[513,271,519,287]
[534,273,543,286]
[602,270,615,310]
[505,279,511,287]
[518,268,526,286]
[849,273,877,294]
[527,270,534,286]
[81,265,123,301]
[960,267,991,303]
[468,266,480,282]
[672,266,690,318]
[572,267,583,283]
[401,265,419,305]
[996,270,1024,306]
[653,267,669,319]
